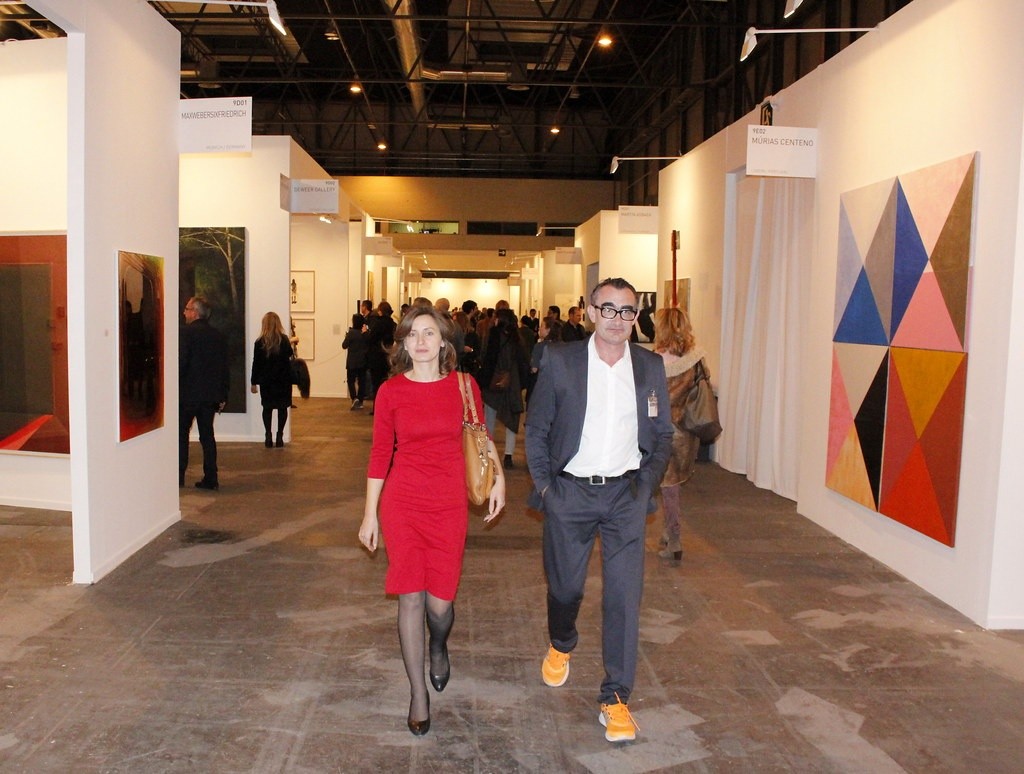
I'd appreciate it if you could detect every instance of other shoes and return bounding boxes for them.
[180,472,185,489]
[350,398,359,411]
[358,404,364,409]
[503,454,515,470]
[660,531,670,545]
[195,473,220,491]
[658,543,683,561]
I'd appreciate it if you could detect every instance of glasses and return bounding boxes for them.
[184,306,195,312]
[591,304,639,321]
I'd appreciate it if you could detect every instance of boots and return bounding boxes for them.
[265,433,273,448]
[276,432,283,447]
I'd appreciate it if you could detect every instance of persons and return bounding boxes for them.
[651,308,710,561]
[342,300,398,416]
[358,304,505,736]
[179,297,230,489]
[401,297,585,468]
[250,312,295,447]
[524,277,674,742]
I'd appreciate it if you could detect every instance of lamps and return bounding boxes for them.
[610,156,682,173]
[740,26,879,59]
[783,0,803,18]
[373,217,414,232]
[536,227,575,237]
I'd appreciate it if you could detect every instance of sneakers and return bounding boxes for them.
[541,643,571,688]
[598,691,641,742]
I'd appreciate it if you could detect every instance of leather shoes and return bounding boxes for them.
[407,690,432,737]
[429,644,451,693]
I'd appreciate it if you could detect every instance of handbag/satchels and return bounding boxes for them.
[681,359,723,445]
[290,354,310,398]
[488,370,515,392]
[457,371,499,506]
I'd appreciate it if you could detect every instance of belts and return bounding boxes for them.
[560,469,644,485]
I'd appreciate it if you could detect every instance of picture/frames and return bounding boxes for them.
[290,270,315,313]
[293,319,315,360]
